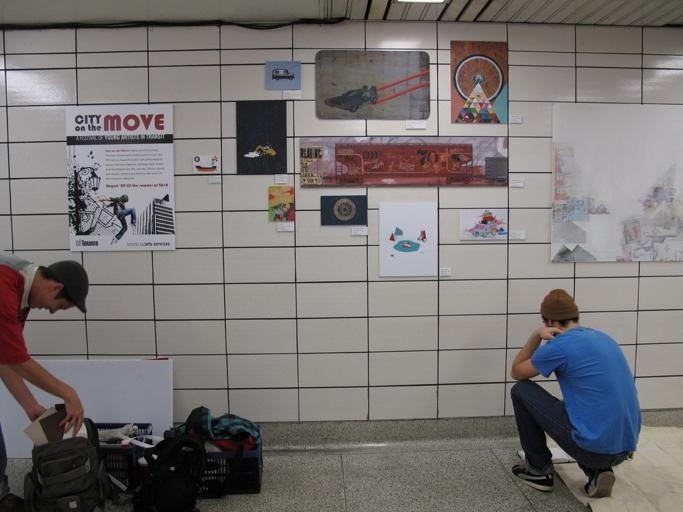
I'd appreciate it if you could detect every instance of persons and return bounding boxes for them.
[507,287,642,499]
[99,194,137,246]
[0,248,88,512]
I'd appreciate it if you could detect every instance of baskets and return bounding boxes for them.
[162,423,264,496]
[91,421,153,490]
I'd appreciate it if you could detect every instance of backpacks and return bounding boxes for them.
[23,400,110,512]
[131,436,199,512]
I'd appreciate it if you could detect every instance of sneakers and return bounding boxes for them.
[511,461,555,493]
[0,493,25,512]
[584,465,617,499]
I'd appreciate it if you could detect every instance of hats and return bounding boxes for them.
[44,258,89,315]
[539,288,579,320]
